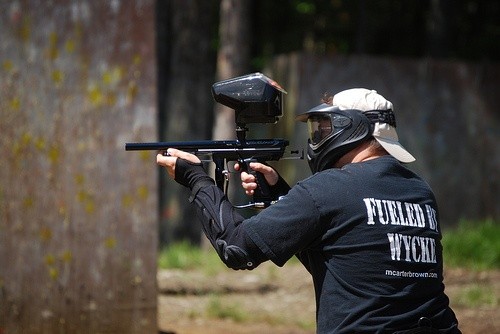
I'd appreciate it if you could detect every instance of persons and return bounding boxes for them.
[156,87,464,334]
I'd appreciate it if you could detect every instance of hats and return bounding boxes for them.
[333,88,416,163]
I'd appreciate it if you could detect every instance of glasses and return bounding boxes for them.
[317,125,331,135]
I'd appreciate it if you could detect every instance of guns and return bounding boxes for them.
[125,73,305,209]
